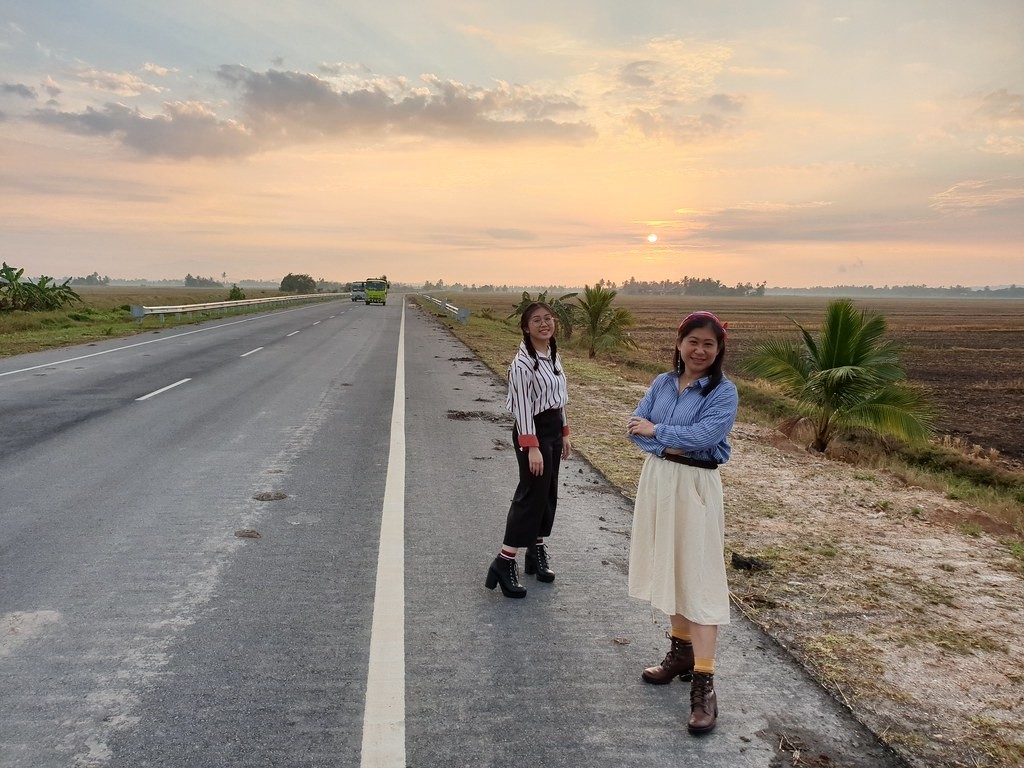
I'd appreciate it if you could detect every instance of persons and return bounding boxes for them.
[484,302,573,598]
[626,311,739,734]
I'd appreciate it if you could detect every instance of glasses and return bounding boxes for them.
[529,317,555,327]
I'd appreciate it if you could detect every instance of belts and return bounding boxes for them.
[656,453,718,469]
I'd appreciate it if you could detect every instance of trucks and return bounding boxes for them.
[349,281,367,302]
[364,278,390,306]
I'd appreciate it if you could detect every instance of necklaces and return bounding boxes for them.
[684,372,691,386]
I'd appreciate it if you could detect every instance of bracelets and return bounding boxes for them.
[652,424,658,437]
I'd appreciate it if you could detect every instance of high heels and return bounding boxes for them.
[525,544,555,582]
[485,553,527,598]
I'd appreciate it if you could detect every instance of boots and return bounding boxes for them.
[642,631,695,685]
[688,671,718,733]
